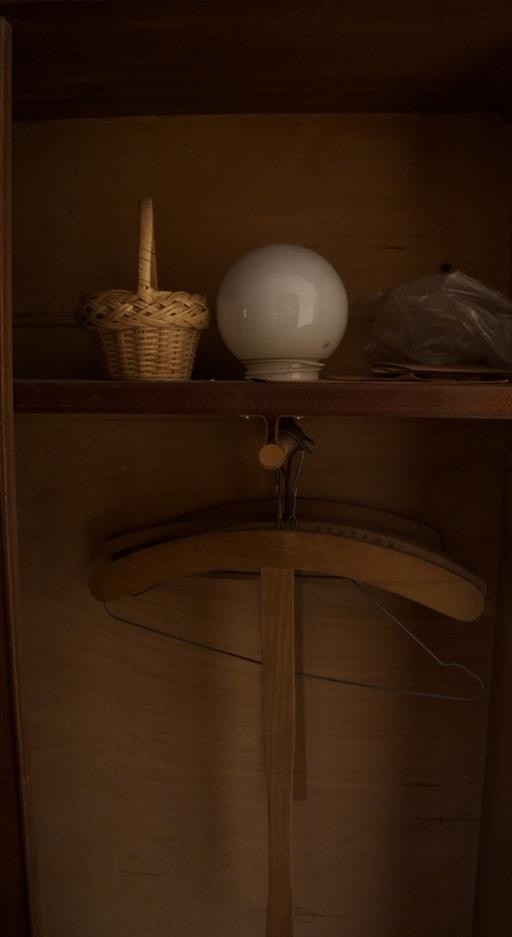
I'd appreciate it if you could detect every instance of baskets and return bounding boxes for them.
[77,195,210,382]
